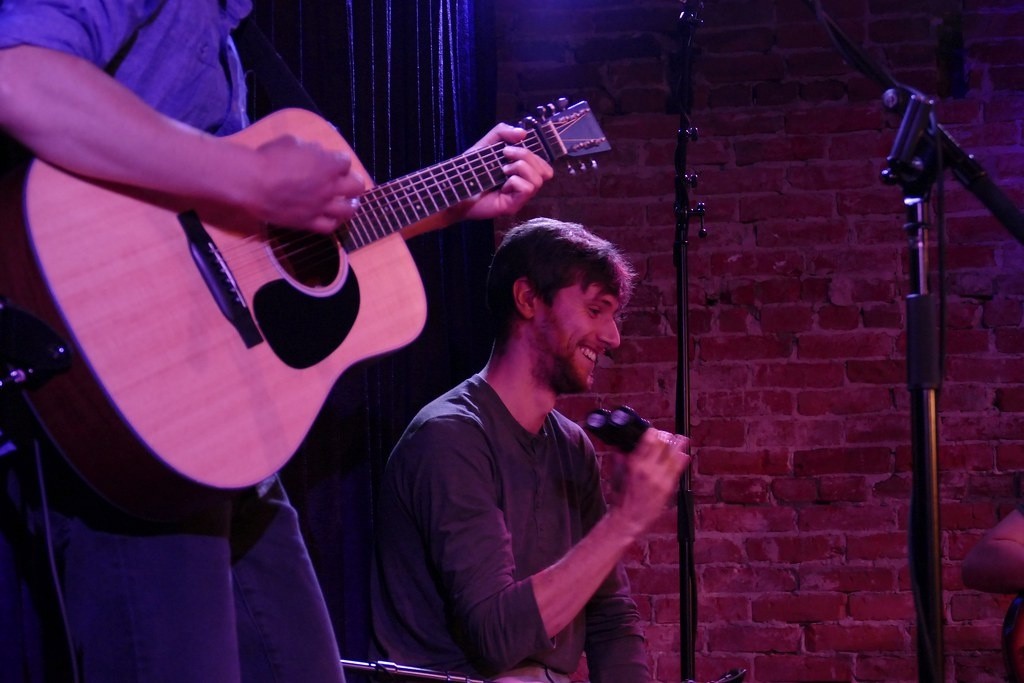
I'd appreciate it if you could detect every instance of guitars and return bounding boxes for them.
[24,92,611,491]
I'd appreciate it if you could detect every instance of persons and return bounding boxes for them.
[959,471,1024,592]
[370,218,691,682]
[1,0,553,683]
